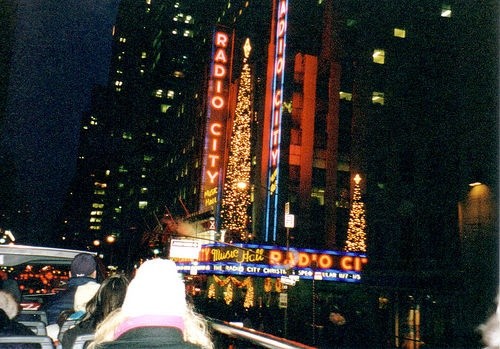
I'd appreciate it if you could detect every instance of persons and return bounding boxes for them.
[0,253,215,349]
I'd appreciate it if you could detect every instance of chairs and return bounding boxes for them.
[0,295,206,349]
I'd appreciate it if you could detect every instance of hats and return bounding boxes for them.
[71,253,97,278]
